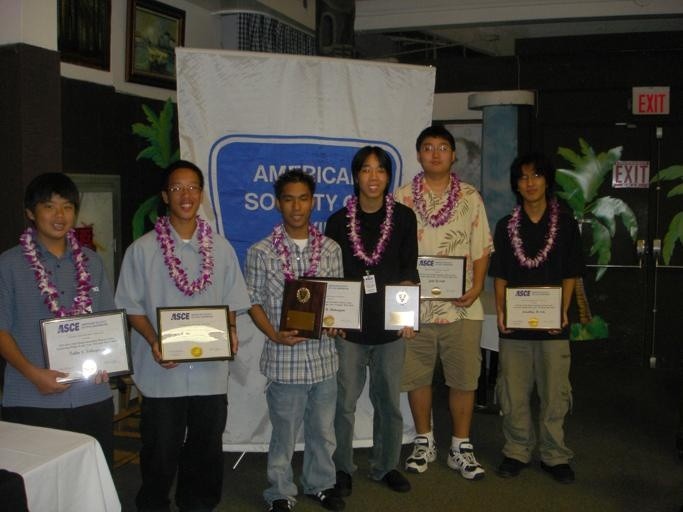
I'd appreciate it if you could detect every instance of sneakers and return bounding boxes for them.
[267,499,293,512]
[495,457,530,477]
[405,436,438,473]
[335,471,352,498]
[307,488,345,512]
[384,469,413,493]
[542,462,575,485]
[446,442,485,481]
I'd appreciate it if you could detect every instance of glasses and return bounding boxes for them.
[165,185,202,194]
[419,145,454,155]
[518,173,544,182]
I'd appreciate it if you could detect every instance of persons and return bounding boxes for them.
[244,168,345,512]
[489,150,580,484]
[114,160,252,511]
[325,144,421,499]
[392,124,495,481]
[0,172,117,465]
